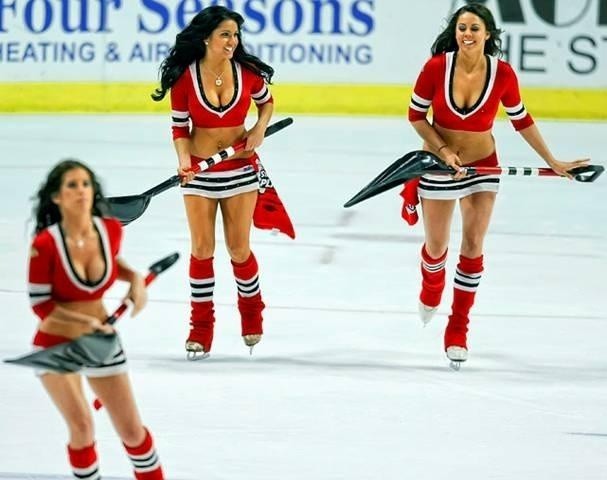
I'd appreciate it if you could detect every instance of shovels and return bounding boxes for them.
[96,117,293,225]
[343,151,604,208]
[4,253,179,373]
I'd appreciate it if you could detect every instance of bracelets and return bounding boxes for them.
[437,143,450,154]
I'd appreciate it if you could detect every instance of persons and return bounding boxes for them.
[152,5,275,359]
[28,158,164,480]
[407,4,590,371]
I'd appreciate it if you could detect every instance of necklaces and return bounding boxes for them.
[201,63,231,86]
[61,225,93,248]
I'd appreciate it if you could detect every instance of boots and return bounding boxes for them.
[125,425,164,479]
[230,251,264,345]
[420,244,447,325]
[444,254,483,362]
[67,444,102,480]
[185,254,215,353]
